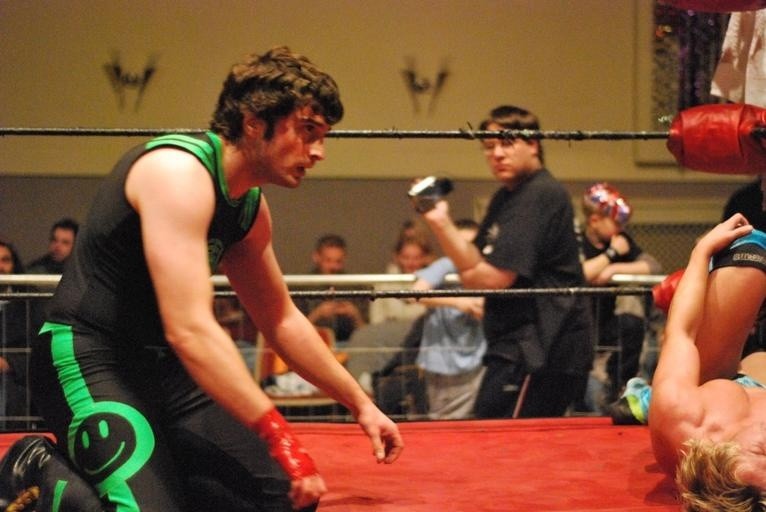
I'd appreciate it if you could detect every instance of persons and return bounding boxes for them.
[0,217,80,431]
[210,215,495,421]
[407,105,593,418]
[1,44,405,511]
[567,100,764,511]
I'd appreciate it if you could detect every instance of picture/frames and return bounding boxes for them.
[635,0,730,164]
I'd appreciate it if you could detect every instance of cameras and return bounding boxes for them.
[408,175,453,215]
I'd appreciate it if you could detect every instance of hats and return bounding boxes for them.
[584,183,632,224]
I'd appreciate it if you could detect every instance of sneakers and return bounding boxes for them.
[1,435,57,512]
[612,377,651,425]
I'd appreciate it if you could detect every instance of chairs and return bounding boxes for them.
[257,329,345,405]
